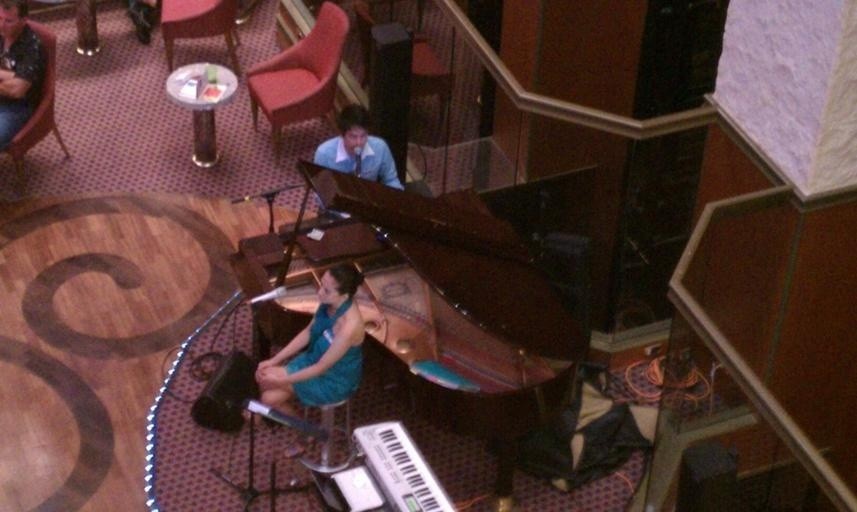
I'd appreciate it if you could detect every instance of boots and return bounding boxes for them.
[128,0,155,44]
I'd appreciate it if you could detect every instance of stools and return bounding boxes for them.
[300,391,353,473]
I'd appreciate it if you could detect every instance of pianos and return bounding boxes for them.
[230,159,592,511]
[310,417,459,511]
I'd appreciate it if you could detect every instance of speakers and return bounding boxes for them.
[191,347,259,433]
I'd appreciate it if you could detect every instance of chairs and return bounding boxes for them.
[358,9,452,148]
[158,0,348,172]
[0,17,68,177]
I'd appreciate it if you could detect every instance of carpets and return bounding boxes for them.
[146,286,658,512]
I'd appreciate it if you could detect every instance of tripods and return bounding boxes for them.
[206,416,313,512]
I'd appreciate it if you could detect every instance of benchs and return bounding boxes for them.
[277,180,435,232]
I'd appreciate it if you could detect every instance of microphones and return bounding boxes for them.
[245,396,332,447]
[246,286,288,307]
[350,145,365,178]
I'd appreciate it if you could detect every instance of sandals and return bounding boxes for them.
[283,437,315,458]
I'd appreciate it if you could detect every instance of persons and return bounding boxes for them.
[254,263,366,458]
[0,0,48,143]
[312,102,406,226]
